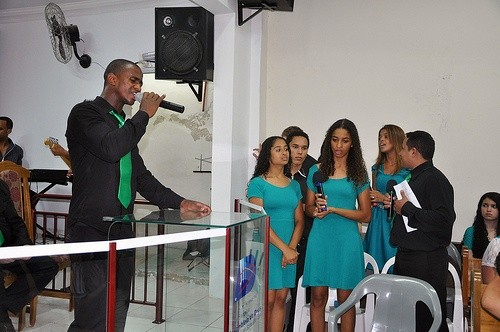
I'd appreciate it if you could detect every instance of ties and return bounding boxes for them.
[112,112,132,209]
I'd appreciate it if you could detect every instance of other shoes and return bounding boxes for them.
[356,308,365,315]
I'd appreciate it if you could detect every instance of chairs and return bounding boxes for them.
[0,160,37,332]
[293,226,469,332]
[183,228,210,271]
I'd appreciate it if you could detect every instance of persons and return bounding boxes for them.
[0,116,24,166]
[301,118,372,332]
[460,192,500,320]
[63,58,212,332]
[45,140,72,291]
[244,125,319,332]
[382,130,457,332]
[359,124,412,309]
[0,177,60,332]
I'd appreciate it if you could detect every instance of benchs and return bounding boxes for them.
[462,245,500,332]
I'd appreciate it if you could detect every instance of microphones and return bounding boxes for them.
[135,92,185,113]
[312,171,327,212]
[386,179,397,222]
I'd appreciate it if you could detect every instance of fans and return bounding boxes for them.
[45,3,92,68]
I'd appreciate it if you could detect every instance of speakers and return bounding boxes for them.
[154,7,213,81]
